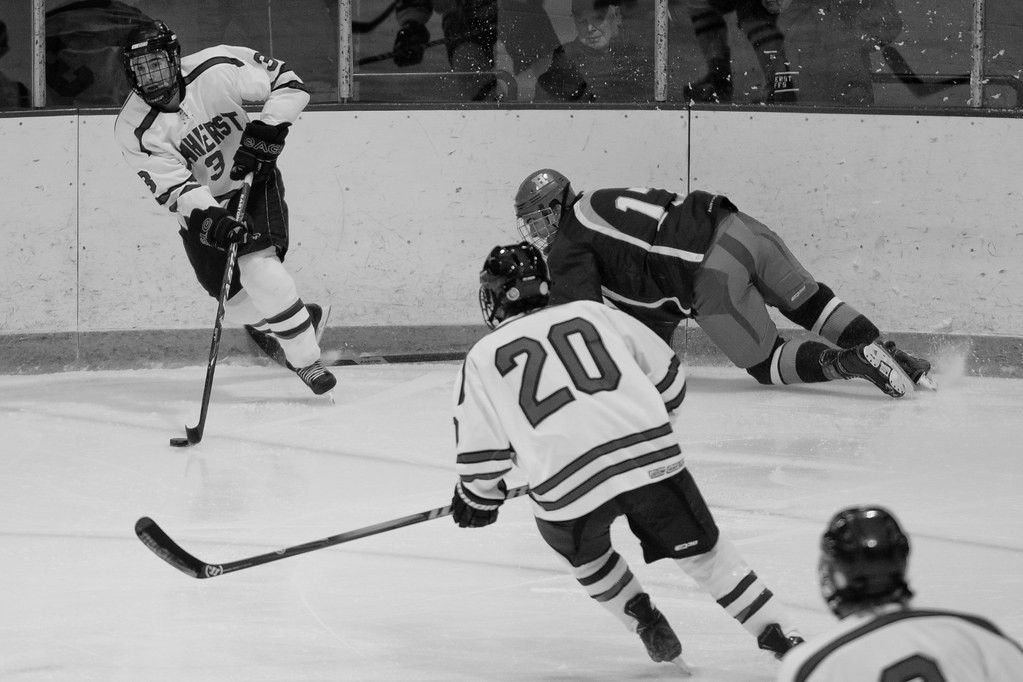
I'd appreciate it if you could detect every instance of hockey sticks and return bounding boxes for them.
[243,323,465,374]
[184,172,255,445]
[882,44,972,97]
[133,483,531,581]
[351,29,475,66]
[353,0,400,34]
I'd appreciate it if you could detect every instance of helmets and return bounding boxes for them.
[512,168,578,219]
[818,504,911,605]
[478,241,551,319]
[119,19,181,108]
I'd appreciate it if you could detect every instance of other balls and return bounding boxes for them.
[169,438,189,447]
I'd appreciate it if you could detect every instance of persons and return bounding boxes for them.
[516,168,937,398]
[450,240,804,671]
[681,0,798,103]
[538,0,657,102]
[763,0,903,106]
[112,19,338,403]
[774,505,1023,682]
[391,0,498,103]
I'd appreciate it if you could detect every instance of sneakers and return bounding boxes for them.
[682,58,735,104]
[756,623,807,663]
[749,55,798,105]
[468,72,504,103]
[624,592,691,677]
[818,340,918,398]
[883,340,938,392]
[286,302,333,373]
[293,358,338,405]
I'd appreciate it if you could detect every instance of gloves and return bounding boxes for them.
[186,206,261,253]
[391,20,431,68]
[229,122,292,185]
[448,482,503,529]
